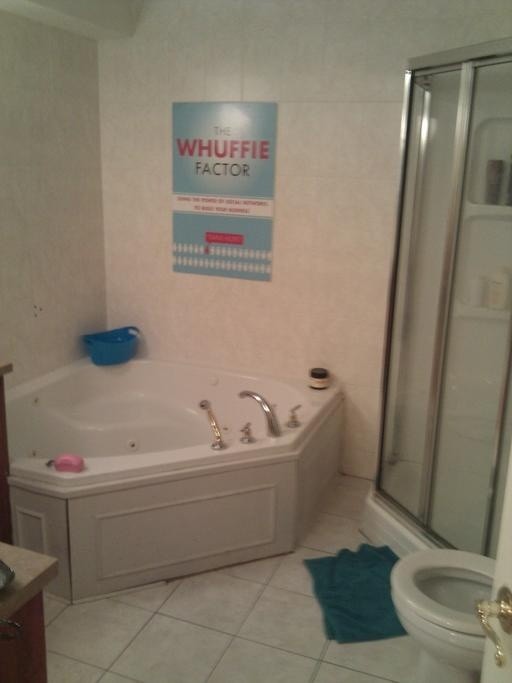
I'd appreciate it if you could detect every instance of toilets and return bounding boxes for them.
[391,548,494,683]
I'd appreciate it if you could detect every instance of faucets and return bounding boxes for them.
[199,399,224,452]
[239,389,281,440]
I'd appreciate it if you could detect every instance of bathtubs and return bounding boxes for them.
[5,355,345,602]
